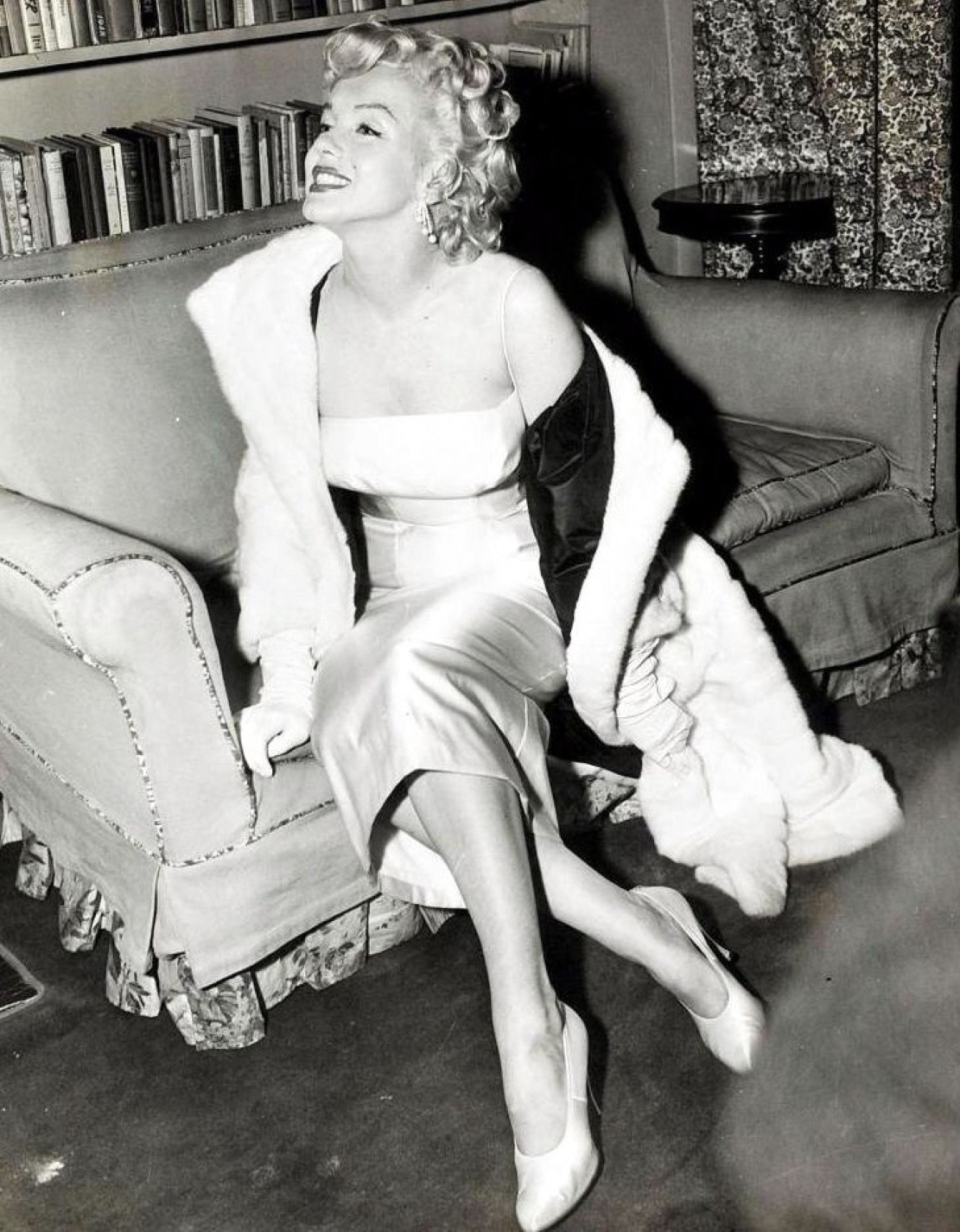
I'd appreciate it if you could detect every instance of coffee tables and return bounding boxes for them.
[648,169,839,283]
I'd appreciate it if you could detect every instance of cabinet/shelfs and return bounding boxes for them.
[0,0,593,257]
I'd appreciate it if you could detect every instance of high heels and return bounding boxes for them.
[506,994,607,1232]
[631,884,772,1076]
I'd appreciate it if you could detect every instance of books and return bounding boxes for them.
[0,1,591,255]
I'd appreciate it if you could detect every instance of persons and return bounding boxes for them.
[185,20,766,1229]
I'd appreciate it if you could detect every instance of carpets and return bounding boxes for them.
[0,930,85,1065]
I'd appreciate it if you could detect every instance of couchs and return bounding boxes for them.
[0,165,959,1056]
